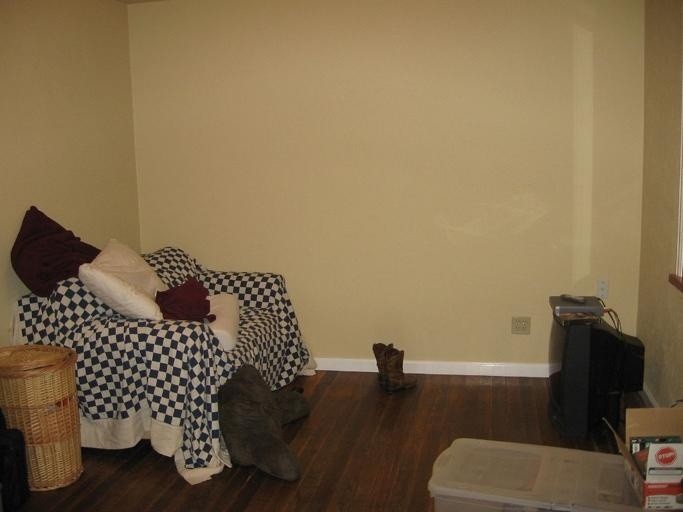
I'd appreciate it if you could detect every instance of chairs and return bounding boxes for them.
[17,245,318,485]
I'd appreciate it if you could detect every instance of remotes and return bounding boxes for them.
[561,293,586,304]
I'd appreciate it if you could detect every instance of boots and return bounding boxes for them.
[372,343,418,393]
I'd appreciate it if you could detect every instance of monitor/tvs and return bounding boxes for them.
[547,313,645,441]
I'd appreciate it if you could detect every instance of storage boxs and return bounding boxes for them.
[426,406,682,512]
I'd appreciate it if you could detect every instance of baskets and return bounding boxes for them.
[0,345,84,493]
